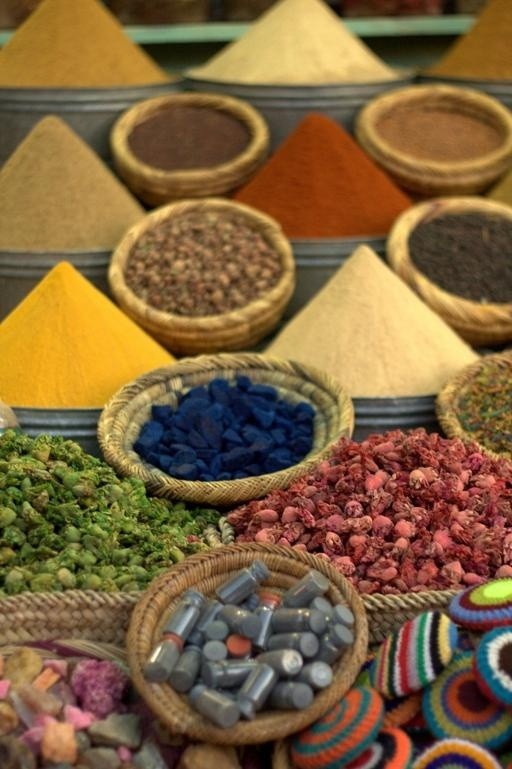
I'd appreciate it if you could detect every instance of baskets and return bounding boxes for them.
[353,82,512,193]
[108,197,297,356]
[107,92,271,206]
[435,347,512,461]
[386,193,512,346]
[99,352,356,505]
[0,514,470,769]
